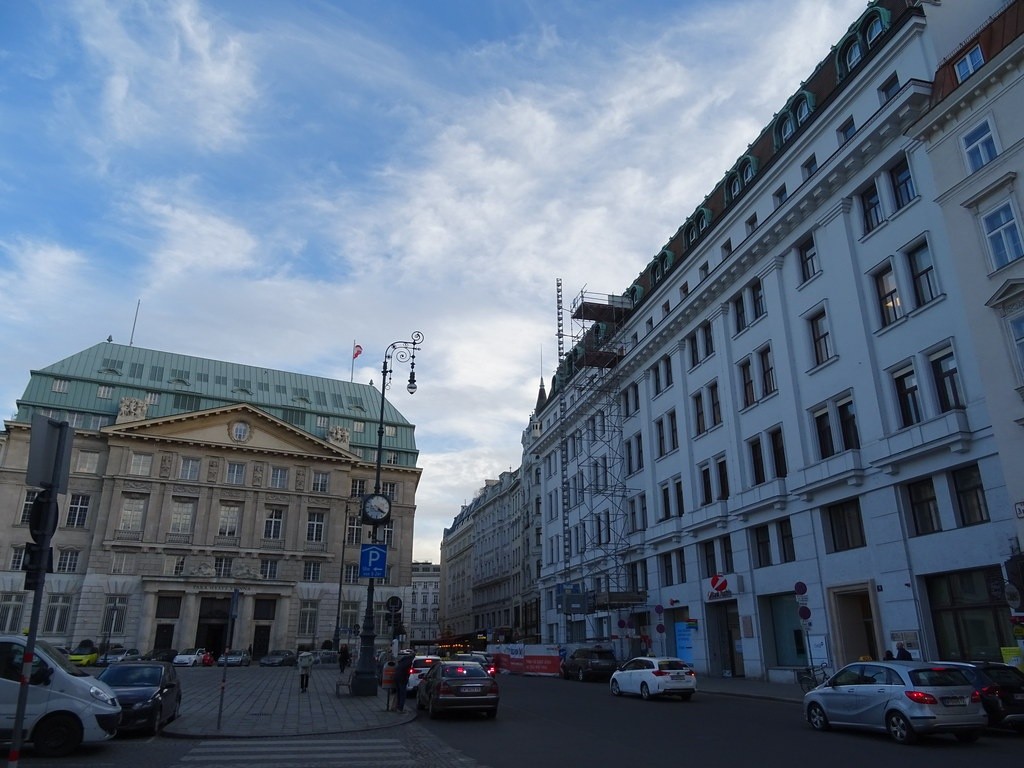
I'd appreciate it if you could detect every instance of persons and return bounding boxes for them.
[249,643,252,664]
[337,644,349,674]
[393,649,416,714]
[298,648,315,694]
[896,642,913,661]
[883,650,896,662]
[555,644,568,680]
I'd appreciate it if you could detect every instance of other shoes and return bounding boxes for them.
[397,708,407,713]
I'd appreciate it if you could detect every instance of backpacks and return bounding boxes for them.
[300,655,312,668]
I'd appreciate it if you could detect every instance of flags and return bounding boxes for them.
[353,345,364,359]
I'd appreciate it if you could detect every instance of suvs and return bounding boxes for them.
[561,648,618,682]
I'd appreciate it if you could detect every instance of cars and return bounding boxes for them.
[415,661,499,718]
[68,647,98,667]
[138,649,178,662]
[94,663,182,738]
[95,648,142,666]
[258,650,296,667]
[218,649,251,667]
[927,661,1024,735]
[0,635,124,758]
[374,649,415,687]
[444,651,495,680]
[803,660,989,744]
[406,655,442,697]
[610,657,697,702]
[172,648,206,667]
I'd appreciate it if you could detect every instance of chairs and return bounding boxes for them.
[336,670,352,696]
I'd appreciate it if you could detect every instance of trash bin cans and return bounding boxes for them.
[382,660,398,711]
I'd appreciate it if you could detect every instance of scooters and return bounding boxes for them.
[203,652,215,667]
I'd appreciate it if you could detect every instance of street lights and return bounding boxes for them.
[349,333,427,693]
[331,507,352,652]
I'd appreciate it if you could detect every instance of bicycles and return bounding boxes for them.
[800,662,830,694]
